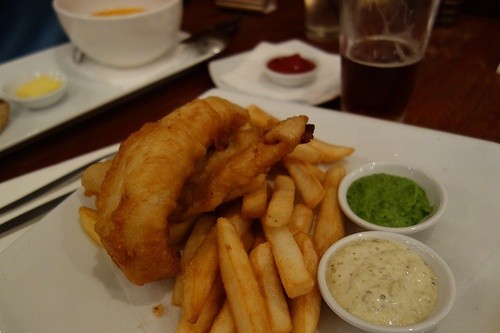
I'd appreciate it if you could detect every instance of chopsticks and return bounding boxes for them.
[0,221,0,238]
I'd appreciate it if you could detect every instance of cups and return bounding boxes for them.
[304,0,339,54]
[339,0,440,123]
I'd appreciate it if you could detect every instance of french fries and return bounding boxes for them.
[76,103,356,333]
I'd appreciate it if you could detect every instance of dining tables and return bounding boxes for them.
[0,0,500,185]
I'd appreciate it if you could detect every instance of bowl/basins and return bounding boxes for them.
[317,231,457,333]
[6,68,68,109]
[262,54,318,88]
[337,160,448,236]
[53,0,182,69]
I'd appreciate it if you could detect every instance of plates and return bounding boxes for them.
[0,29,225,152]
[0,88,500,333]
[209,39,341,105]
